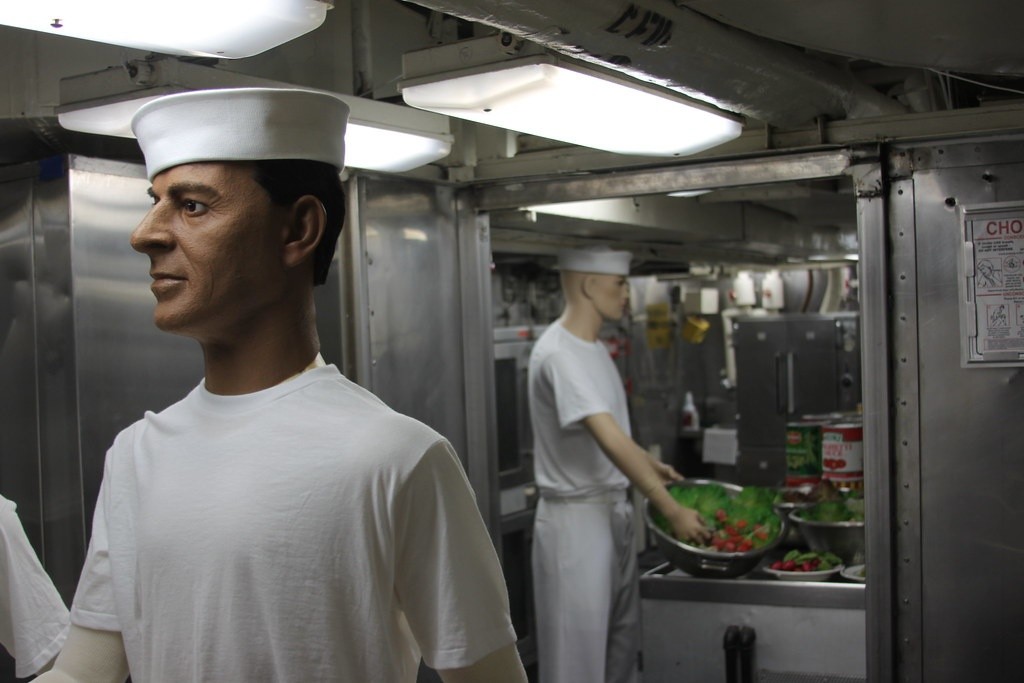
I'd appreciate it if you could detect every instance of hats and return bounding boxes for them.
[130,87,350,183]
[557,242,634,276]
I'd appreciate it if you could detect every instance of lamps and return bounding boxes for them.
[0,2,335,62]
[394,26,747,162]
[54,56,456,175]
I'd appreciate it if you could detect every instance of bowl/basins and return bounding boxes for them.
[788,509,865,565]
[643,480,785,579]
[774,486,851,520]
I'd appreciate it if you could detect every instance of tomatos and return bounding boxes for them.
[771,558,821,572]
[710,508,768,552]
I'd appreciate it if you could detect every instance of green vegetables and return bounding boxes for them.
[649,480,782,548]
[784,488,864,571]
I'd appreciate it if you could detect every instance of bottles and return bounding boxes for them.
[681,392,700,432]
[732,271,757,306]
[761,273,785,309]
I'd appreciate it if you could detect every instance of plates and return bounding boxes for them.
[761,564,844,581]
[840,564,866,582]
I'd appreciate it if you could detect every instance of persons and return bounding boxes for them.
[0,495,72,676]
[526,246,711,683]
[26,87,529,683]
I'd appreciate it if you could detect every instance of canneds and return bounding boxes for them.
[785,412,865,496]
